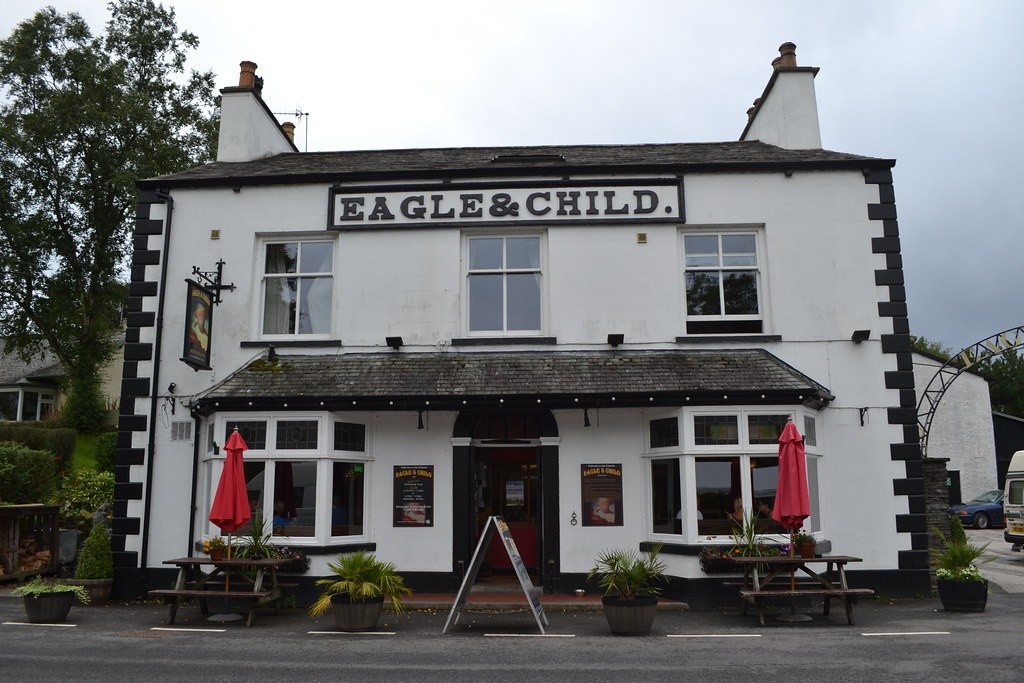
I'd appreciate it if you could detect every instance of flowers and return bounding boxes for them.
[281,546,302,561]
[699,511,791,559]
[202,537,228,554]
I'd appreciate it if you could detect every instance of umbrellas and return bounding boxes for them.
[772,417,810,614]
[208,425,251,615]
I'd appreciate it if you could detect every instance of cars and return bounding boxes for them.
[949,489,1006,530]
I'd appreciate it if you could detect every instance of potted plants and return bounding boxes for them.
[307,550,416,632]
[584,542,672,636]
[926,515,997,612]
[10,574,92,624]
[793,534,815,558]
[72,520,113,604]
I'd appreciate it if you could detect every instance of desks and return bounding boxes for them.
[730,555,863,625]
[163,558,292,626]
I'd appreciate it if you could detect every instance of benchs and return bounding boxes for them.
[738,589,874,626]
[172,581,299,615]
[147,589,271,627]
[723,579,845,615]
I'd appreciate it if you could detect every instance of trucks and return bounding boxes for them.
[999,449,1024,555]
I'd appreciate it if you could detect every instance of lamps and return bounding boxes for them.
[386,337,403,349]
[851,329,871,344]
[607,334,624,347]
[520,464,537,481]
[168,382,176,393]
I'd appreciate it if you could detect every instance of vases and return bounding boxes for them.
[210,552,223,561]
